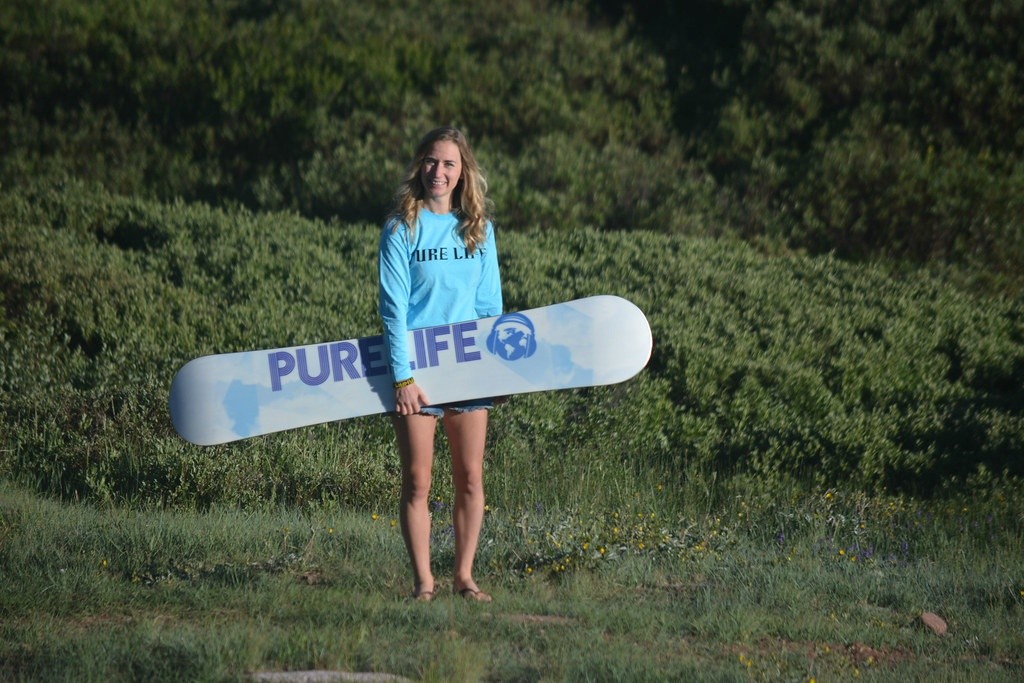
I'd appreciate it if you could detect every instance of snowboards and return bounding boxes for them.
[168,293,653,447]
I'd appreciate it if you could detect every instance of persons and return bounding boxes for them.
[379,127,503,602]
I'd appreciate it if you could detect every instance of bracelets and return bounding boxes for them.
[393,377,414,389]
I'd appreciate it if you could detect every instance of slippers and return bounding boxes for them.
[459,588,493,603]
[413,590,436,602]
